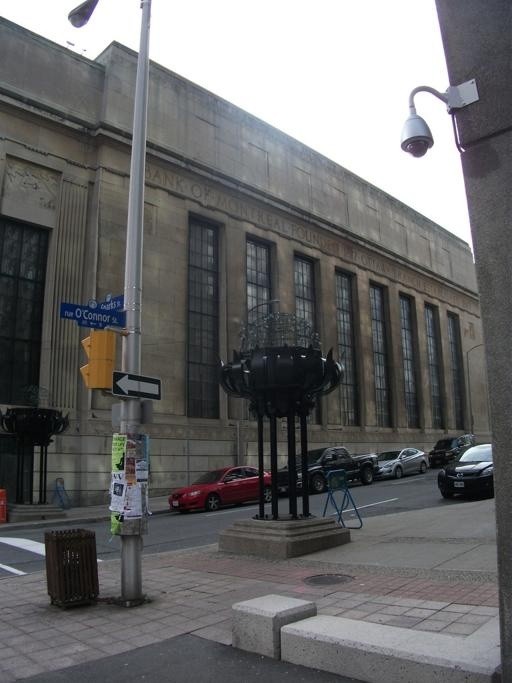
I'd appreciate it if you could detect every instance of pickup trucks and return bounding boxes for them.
[271,440,379,495]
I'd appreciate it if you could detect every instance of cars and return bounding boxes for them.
[436,440,495,501]
[368,446,431,479]
[167,464,278,514]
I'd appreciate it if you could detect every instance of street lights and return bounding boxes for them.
[236,298,281,465]
[65,0,153,611]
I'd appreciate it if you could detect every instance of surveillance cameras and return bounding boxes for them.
[399,77,478,159]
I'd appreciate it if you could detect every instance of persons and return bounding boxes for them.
[115,483,121,494]
[115,451,125,470]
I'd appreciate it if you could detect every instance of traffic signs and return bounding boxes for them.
[76,293,125,326]
[59,301,126,327]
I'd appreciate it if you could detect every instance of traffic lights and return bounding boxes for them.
[78,326,116,390]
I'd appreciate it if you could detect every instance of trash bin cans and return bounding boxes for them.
[46,527,99,609]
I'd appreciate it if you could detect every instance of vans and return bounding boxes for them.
[427,433,474,466]
